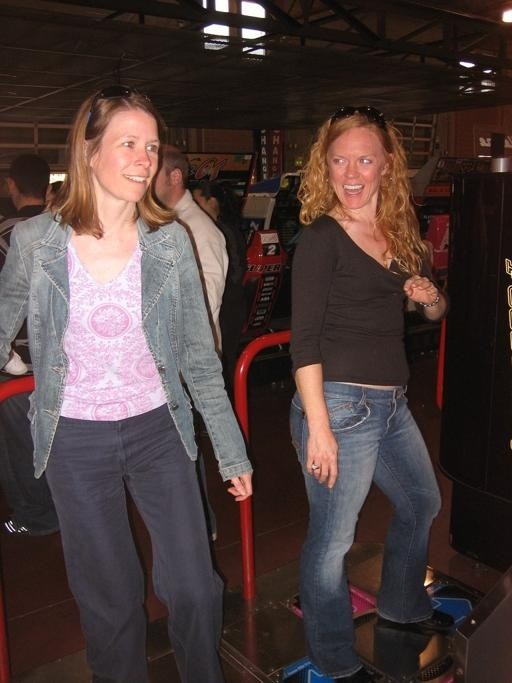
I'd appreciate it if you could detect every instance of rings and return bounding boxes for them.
[312,464,319,470]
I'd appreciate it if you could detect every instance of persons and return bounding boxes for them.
[0,152,65,536]
[289,106,455,683]
[1,85,253,683]
[150,145,254,538]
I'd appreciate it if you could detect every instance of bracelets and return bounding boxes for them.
[420,295,440,308]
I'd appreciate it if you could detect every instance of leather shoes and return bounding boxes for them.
[334,667,373,683]
[379,609,454,629]
